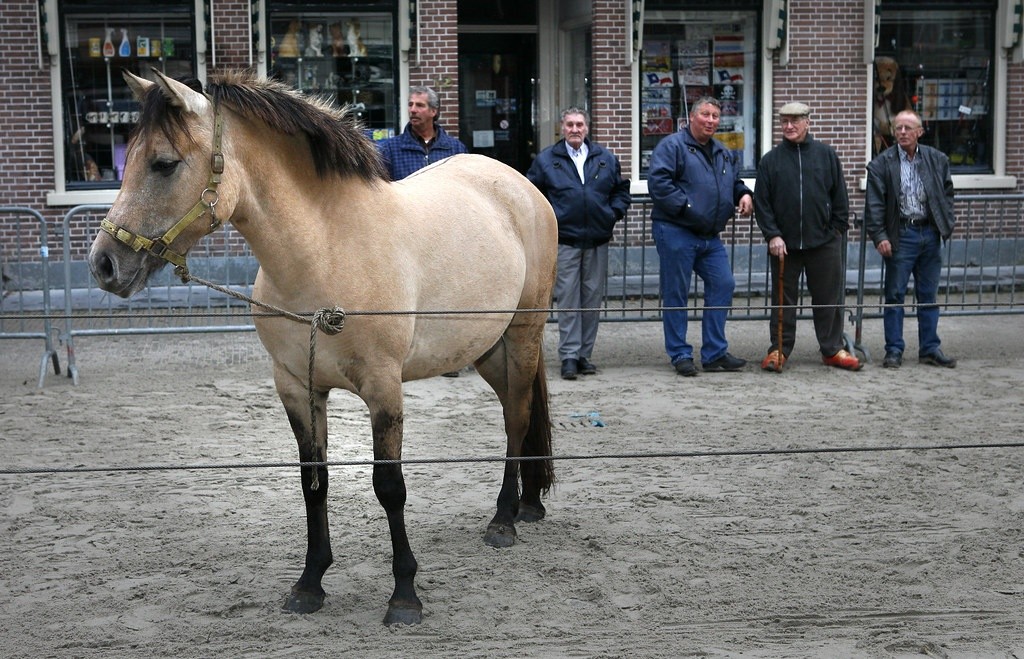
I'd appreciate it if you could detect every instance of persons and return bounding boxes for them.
[374,86,468,181]
[752,101,861,372]
[864,109,957,369]
[526,106,632,380]
[648,96,754,377]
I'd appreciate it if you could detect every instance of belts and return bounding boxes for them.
[901,218,934,226]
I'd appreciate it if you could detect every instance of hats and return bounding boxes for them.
[779,102,810,116]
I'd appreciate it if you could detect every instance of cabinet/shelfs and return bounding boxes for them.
[279,53,392,127]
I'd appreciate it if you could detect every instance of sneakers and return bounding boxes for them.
[675,358,698,376]
[762,349,786,370]
[822,349,863,370]
[703,352,746,369]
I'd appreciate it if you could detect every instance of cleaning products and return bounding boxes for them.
[119,28,131,57]
[103,28,116,57]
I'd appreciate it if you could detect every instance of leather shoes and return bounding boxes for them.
[883,350,902,368]
[561,358,578,378]
[578,356,596,374]
[919,348,956,367]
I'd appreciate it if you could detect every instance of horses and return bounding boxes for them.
[86,66,559,629]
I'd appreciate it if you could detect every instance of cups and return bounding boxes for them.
[89,38,100,57]
[164,38,174,56]
[151,41,161,56]
